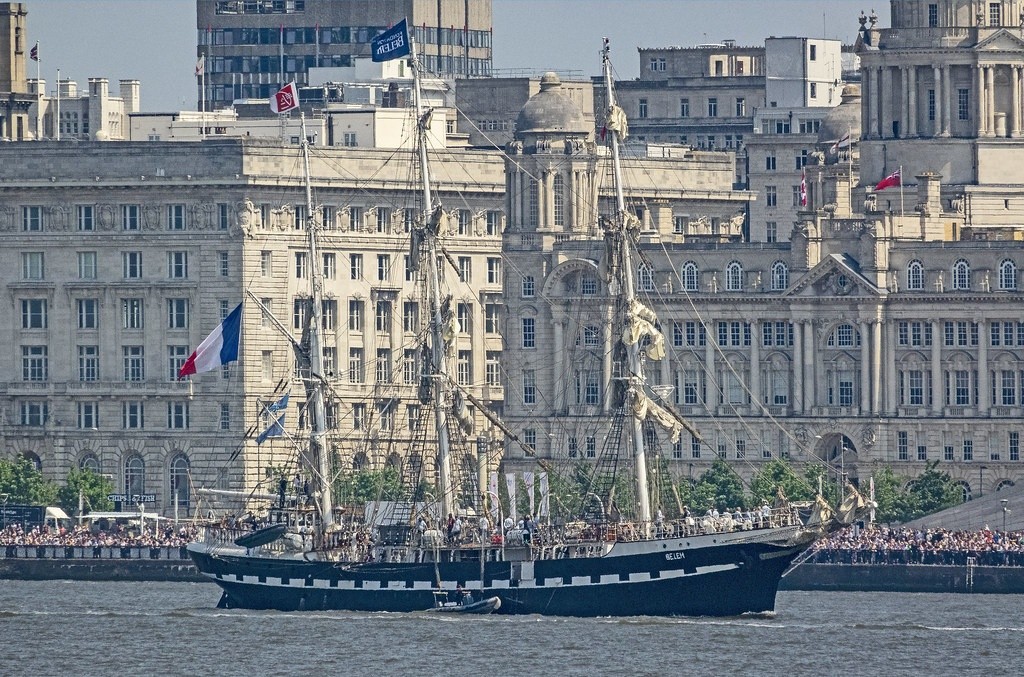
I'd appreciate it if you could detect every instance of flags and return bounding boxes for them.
[800,171,806,206]
[192,56,204,77]
[538,472,548,517]
[369,19,409,62]
[875,167,902,191]
[30,43,38,61]
[267,392,288,411]
[270,82,299,115]
[489,473,497,517]
[255,414,284,445]
[505,472,515,519]
[179,301,243,379]
[523,472,534,516]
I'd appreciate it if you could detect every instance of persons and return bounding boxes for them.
[446,513,540,546]
[278,475,287,508]
[803,524,1024,567]
[0,522,197,548]
[411,516,428,534]
[292,473,300,493]
[652,505,667,539]
[304,477,310,494]
[681,503,773,538]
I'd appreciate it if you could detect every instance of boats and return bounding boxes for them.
[0,542,217,583]
[425,591,502,615]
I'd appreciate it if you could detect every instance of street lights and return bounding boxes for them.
[814,436,829,500]
[688,463,697,509]
[91,427,103,513]
[548,433,561,497]
[1000,498,1010,544]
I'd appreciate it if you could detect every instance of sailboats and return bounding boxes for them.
[184,16,880,618]
